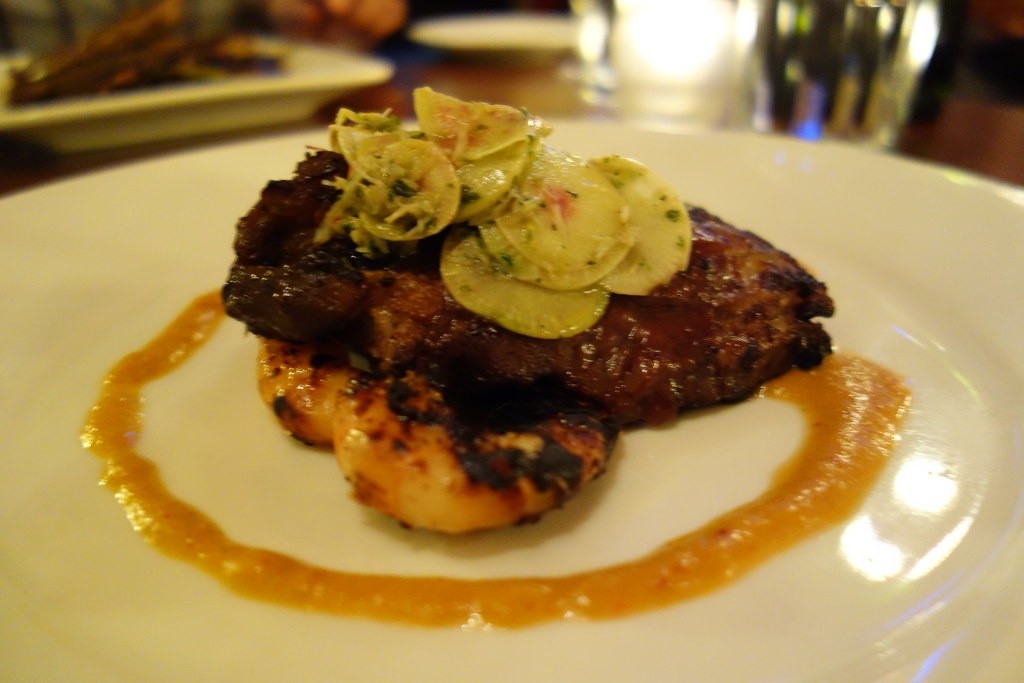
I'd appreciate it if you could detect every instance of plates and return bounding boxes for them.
[0,36,394,148]
[0,117,1024,683]
[412,17,573,51]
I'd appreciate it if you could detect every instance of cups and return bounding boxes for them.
[570,0,764,129]
[764,0,941,147]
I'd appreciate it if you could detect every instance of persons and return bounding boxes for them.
[0,0,407,58]
